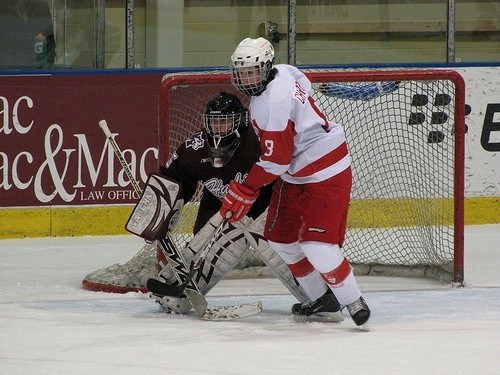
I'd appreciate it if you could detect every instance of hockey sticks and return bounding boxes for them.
[146,209,232,298]
[99,119,262,321]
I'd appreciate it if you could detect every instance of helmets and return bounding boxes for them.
[230,38,275,96]
[201,92,249,168]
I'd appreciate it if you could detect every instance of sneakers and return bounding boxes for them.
[346,295,372,331]
[291,283,343,322]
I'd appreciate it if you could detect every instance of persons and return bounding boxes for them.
[145,92,341,322]
[219,35,369,327]
[0,0,55,70]
